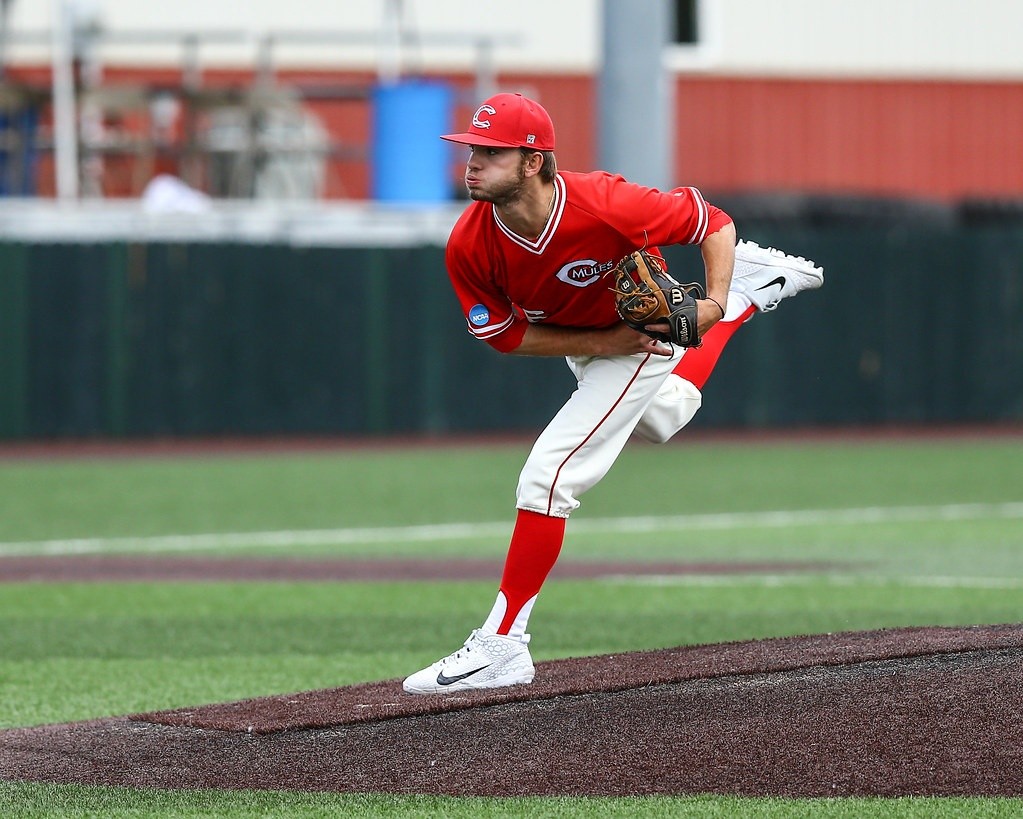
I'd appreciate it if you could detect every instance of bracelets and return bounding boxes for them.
[703,293,726,322]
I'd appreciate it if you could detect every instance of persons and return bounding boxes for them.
[402,93,824,693]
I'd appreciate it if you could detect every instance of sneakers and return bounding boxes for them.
[402,628,537,693]
[728,238,825,313]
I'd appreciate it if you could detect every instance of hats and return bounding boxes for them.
[439,91,556,154]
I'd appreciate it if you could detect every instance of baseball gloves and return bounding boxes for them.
[614,249,701,348]
